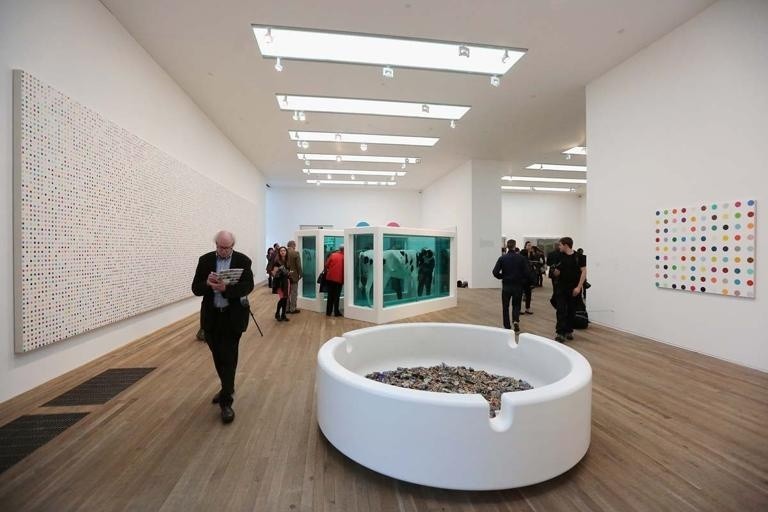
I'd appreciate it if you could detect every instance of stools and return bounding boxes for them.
[214,304,230,314]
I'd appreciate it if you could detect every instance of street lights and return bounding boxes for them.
[573,302,590,329]
[316,266,328,293]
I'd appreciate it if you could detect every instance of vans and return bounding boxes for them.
[274,312,291,322]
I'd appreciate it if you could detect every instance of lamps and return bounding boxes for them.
[299,177,399,189]
[300,167,409,178]
[275,93,472,128]
[297,151,423,169]
[251,23,529,86]
[287,129,441,151]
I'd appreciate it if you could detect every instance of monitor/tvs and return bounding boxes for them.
[215,268,243,293]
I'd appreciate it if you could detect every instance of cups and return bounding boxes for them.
[216,242,234,252]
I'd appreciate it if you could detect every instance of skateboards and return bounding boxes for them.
[358,247,436,308]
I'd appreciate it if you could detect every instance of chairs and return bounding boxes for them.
[555,334,574,343]
[285,309,301,315]
[519,310,534,315]
[513,321,520,332]
[326,311,344,317]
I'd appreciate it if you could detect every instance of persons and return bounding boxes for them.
[323,244,343,316]
[418,247,435,296]
[264,240,303,322]
[515,236,585,315]
[550,236,585,343]
[190,230,254,422]
[491,239,529,331]
[501,248,506,255]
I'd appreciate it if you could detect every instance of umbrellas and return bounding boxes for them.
[219,404,235,425]
[212,389,224,405]
